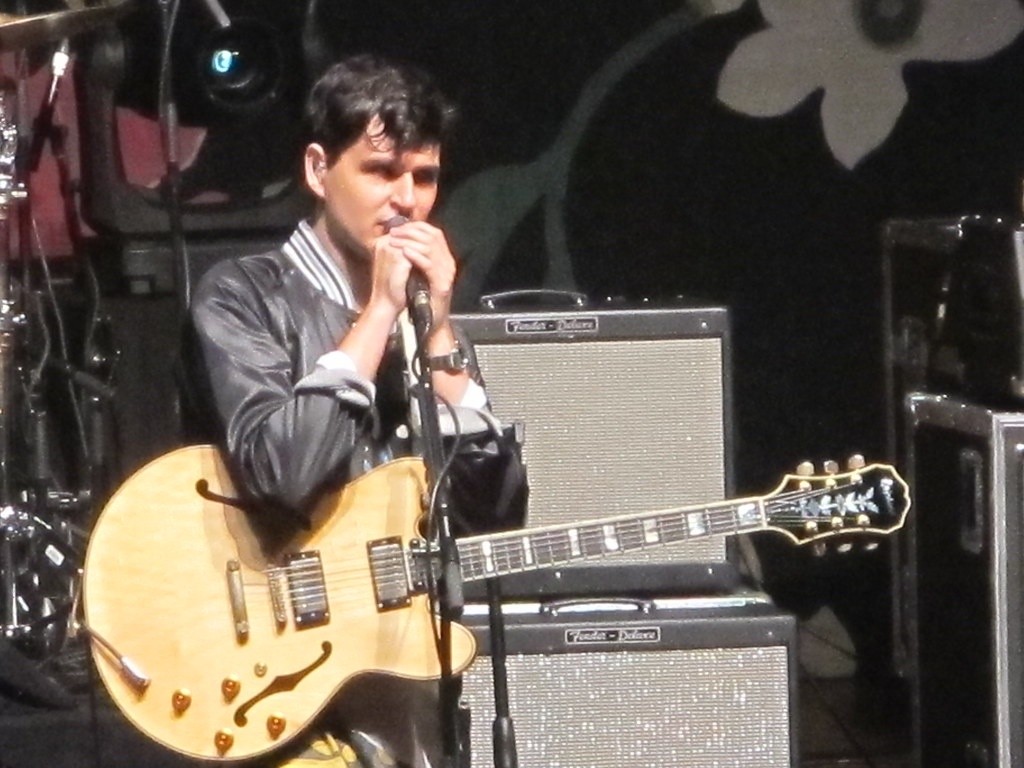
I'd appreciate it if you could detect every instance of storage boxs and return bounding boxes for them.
[905,390,1024,768]
[879,214,1024,679]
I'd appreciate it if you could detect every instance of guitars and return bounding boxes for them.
[81,435,913,765]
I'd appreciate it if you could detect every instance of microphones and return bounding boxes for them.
[383,214,433,326]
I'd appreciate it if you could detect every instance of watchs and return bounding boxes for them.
[431,341,469,372]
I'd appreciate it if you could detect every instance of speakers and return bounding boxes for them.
[439,305,743,600]
[458,591,804,768]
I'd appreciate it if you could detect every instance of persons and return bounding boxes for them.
[181,54,529,768]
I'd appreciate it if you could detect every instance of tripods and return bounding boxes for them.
[0,93,92,649]
